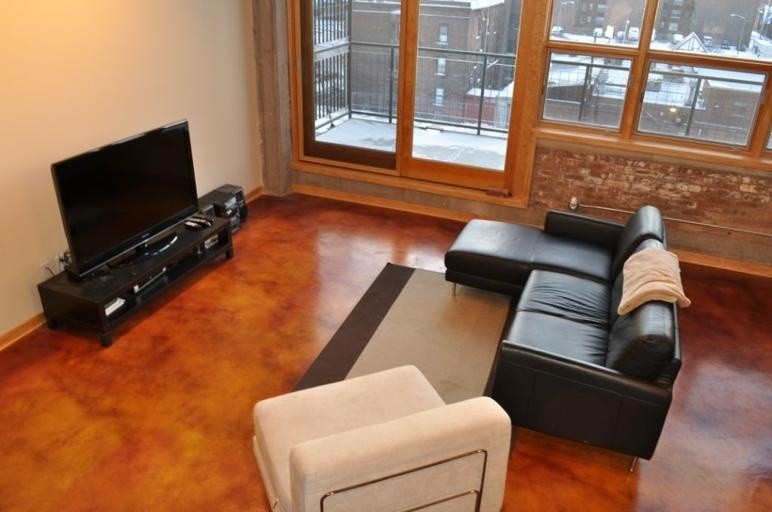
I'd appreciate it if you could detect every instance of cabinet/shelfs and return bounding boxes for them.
[35,214,234,348]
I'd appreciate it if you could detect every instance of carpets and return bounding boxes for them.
[287,258,512,415]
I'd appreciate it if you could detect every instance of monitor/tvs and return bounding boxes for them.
[49,118,200,277]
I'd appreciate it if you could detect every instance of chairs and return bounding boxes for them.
[251,361,514,512]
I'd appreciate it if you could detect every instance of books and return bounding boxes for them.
[106,298,127,316]
